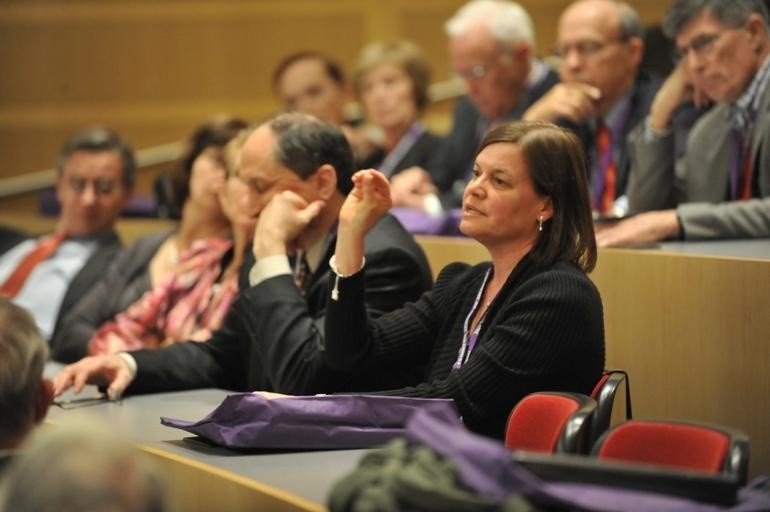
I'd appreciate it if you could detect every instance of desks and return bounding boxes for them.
[1,200,770,480]
[0,383,766,510]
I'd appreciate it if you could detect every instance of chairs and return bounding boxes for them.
[586,369,635,430]
[501,391,598,462]
[591,418,748,481]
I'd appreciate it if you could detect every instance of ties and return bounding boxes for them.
[0,228,70,297]
[597,126,617,215]
[739,105,754,191]
